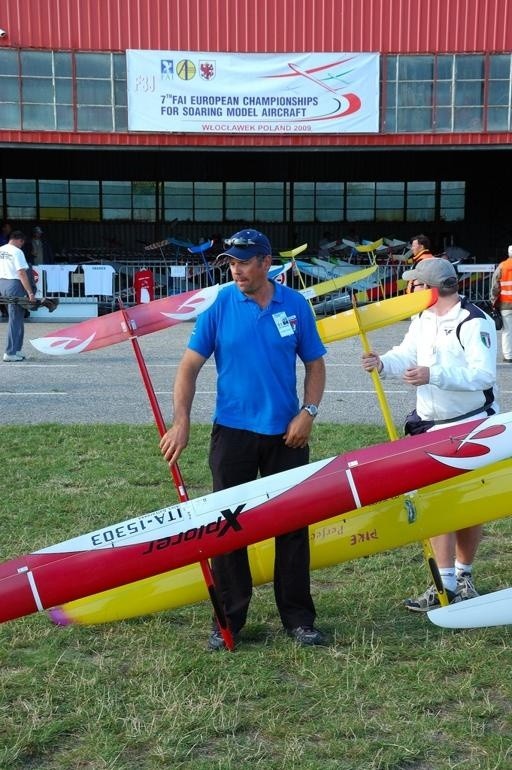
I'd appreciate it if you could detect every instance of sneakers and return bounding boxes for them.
[3,353,24,361]
[404,572,479,611]
[208,630,227,649]
[289,626,327,645]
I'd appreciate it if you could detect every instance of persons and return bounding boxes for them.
[405,236,434,320]
[0,231,37,362]
[362,258,499,611]
[490,244,511,364]
[159,228,326,650]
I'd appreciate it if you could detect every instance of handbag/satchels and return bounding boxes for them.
[492,310,503,330]
[404,409,432,436]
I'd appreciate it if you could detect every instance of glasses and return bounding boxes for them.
[223,237,266,250]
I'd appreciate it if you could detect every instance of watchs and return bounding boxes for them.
[301,404,319,417]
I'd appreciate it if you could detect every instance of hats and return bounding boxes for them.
[216,229,272,262]
[401,258,458,288]
